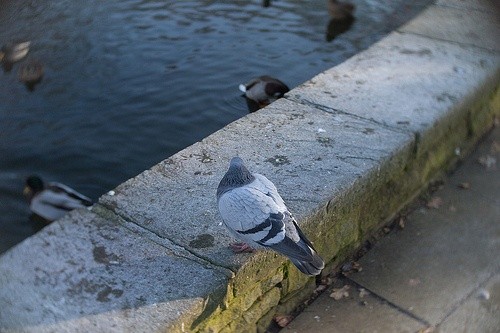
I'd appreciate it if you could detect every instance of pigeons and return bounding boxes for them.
[215,156,325,277]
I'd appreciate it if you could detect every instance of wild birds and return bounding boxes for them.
[24,175,97,221]
[327,0,354,20]
[0,40,31,64]
[238,74,290,109]
[18,50,47,82]
